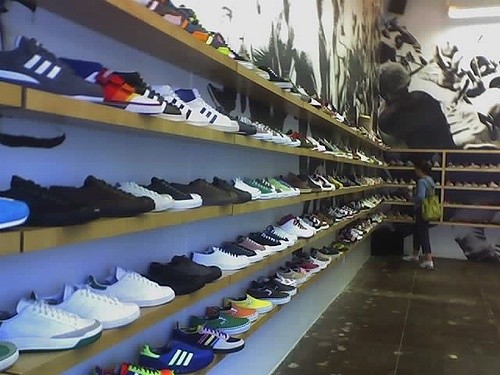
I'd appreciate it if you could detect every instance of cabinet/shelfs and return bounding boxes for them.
[0,0,500,375]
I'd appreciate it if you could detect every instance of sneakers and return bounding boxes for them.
[85,265,175,308]
[33,284,139,329]
[91,361,175,375]
[170,320,245,351]
[206,303,257,322]
[0,175,101,226]
[114,181,173,212]
[171,169,500,232]
[0,35,104,103]
[0,291,102,352]
[50,174,155,216]
[136,0,389,150]
[60,35,500,172]
[139,339,214,374]
[139,177,203,209]
[248,194,500,375]
[191,311,251,335]
[223,291,272,313]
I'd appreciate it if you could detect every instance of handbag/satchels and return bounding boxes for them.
[422,195,442,222]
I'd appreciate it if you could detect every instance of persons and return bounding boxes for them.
[403,160,434,270]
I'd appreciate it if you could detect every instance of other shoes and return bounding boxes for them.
[403,255,419,261]
[0,197,30,230]
[0,342,19,372]
[420,260,434,268]
[144,234,272,297]
